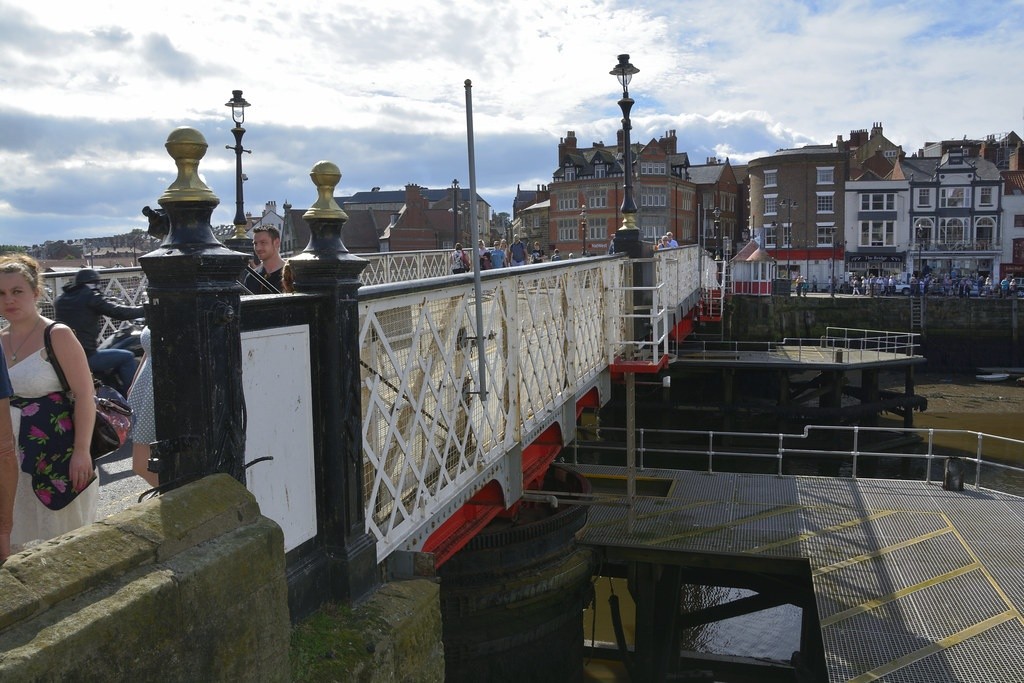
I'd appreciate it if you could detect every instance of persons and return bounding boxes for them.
[53,268,146,393]
[658,232,679,249]
[478,233,528,269]
[908,272,972,299]
[125,326,159,489]
[449,242,470,274]
[552,249,561,261]
[0,336,20,563]
[976,276,1017,299]
[530,242,546,263]
[827,275,840,294]
[850,273,898,296]
[811,275,817,292]
[795,275,810,297]
[0,254,100,549]
[244,224,287,295]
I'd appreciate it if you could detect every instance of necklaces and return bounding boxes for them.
[8,317,40,361]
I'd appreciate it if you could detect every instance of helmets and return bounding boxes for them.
[75,269,101,286]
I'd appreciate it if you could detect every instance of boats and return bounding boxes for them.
[975,373,1009,382]
[974,366,1024,374]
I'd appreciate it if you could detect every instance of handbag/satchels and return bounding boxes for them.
[45,324,132,461]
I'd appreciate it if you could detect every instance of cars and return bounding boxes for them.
[1013,277,1024,297]
[952,277,991,297]
[895,281,926,297]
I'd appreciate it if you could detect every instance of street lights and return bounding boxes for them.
[712,207,722,267]
[579,204,587,255]
[917,228,925,279]
[607,54,642,257]
[450,177,460,250]
[778,193,799,280]
[224,90,252,240]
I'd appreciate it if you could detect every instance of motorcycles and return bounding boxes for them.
[95,290,151,359]
